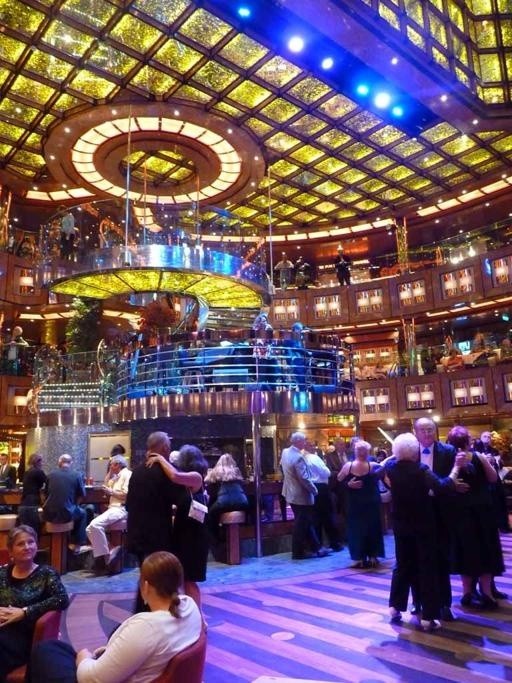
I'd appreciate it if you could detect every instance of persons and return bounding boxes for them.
[7,207,449,291]
[0,311,511,403]
[381,417,509,630]
[280,432,388,569]
[23,551,202,683]
[1,442,133,570]
[1,525,70,683]
[124,431,249,634]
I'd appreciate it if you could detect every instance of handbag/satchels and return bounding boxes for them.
[189,500,207,523]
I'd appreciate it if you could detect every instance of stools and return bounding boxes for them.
[220,511,245,564]
[179,368,204,394]
[105,520,128,573]
[45,521,75,575]
[0,514,18,531]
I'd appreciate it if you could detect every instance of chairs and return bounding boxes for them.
[150,628,207,683]
[7,609,61,683]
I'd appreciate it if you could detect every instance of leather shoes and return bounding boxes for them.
[388,589,508,631]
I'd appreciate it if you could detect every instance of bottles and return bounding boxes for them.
[83,476,87,484]
[88,476,95,485]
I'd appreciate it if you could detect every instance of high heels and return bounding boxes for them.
[369,558,378,568]
[350,560,364,568]
